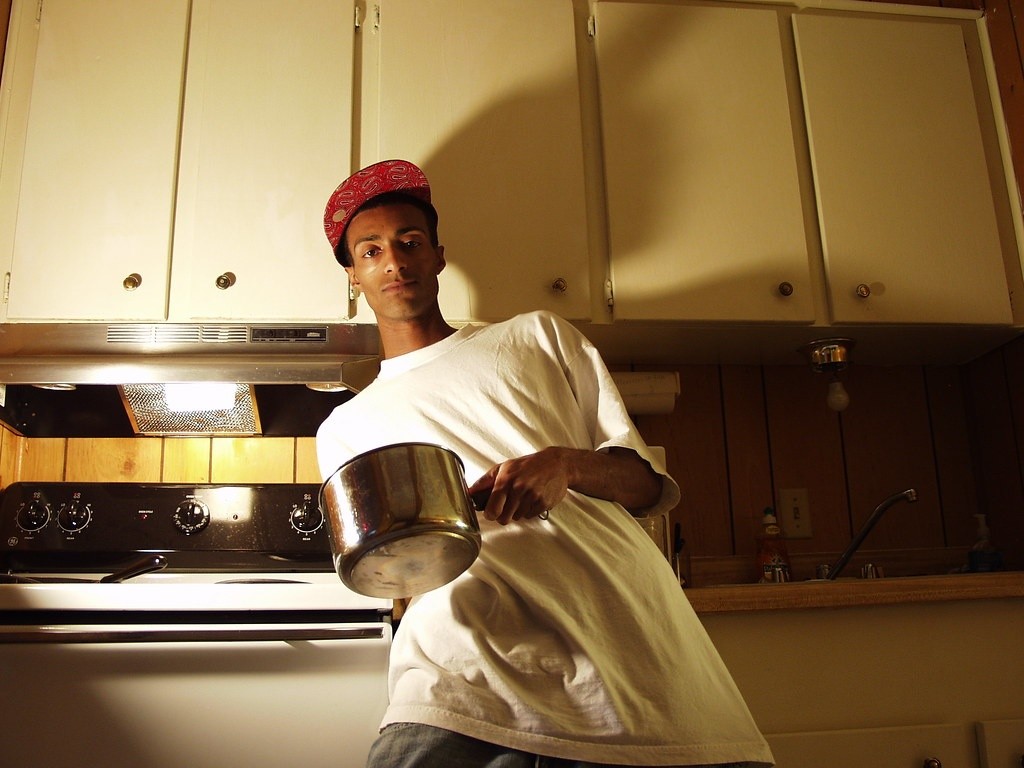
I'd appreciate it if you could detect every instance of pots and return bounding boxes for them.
[319,445,510,599]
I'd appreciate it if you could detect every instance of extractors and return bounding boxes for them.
[0,324,383,439]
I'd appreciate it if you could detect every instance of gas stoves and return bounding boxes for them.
[1,480,396,613]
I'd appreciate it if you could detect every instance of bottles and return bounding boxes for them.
[740,507,790,583]
[969,513,1001,571]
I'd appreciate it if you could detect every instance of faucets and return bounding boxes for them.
[816,487,919,580]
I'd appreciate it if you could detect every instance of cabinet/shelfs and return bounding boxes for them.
[0,0,1024,342]
[696,597,1024,768]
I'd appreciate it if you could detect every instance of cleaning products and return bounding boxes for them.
[967,514,1003,571]
[752,507,792,584]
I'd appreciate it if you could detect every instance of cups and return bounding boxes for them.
[672,551,692,588]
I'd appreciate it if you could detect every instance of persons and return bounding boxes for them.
[314,160,777,768]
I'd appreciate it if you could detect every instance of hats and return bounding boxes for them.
[324,160,431,268]
